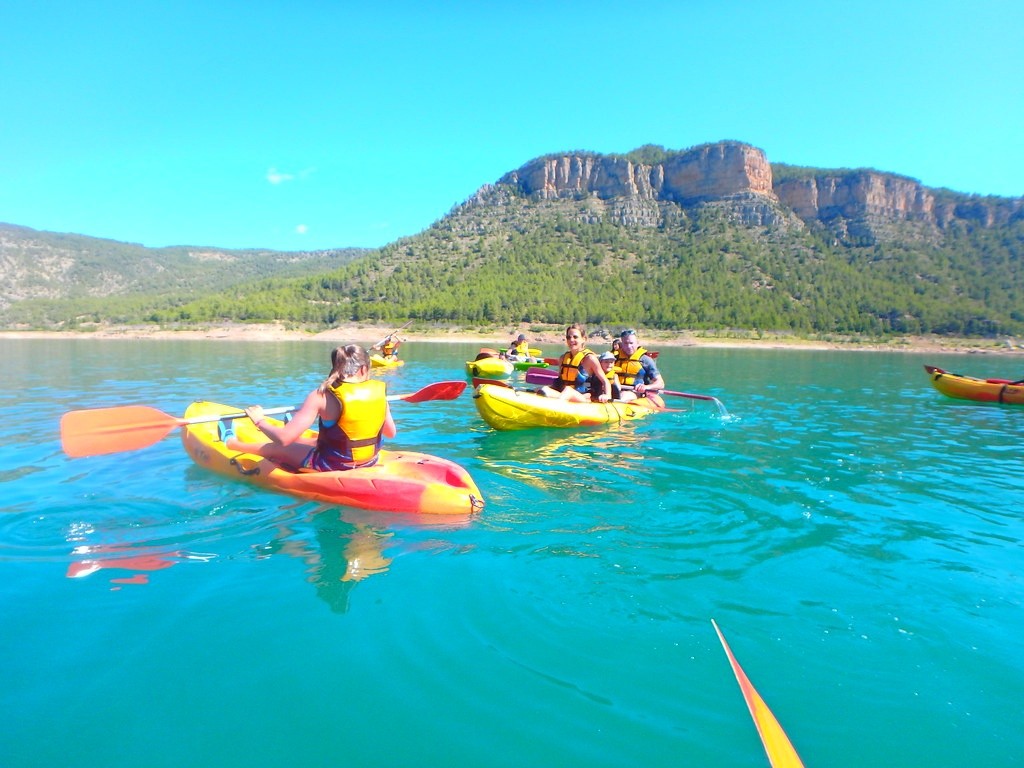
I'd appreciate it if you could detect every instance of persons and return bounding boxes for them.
[505,334,536,364]
[537,325,664,403]
[218,345,396,471]
[372,332,401,361]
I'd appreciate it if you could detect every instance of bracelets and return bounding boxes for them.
[255,418,264,431]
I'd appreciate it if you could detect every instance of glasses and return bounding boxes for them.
[566,335,582,339]
[622,330,635,339]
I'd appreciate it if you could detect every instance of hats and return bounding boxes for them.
[519,334,525,340]
[599,352,616,363]
[385,335,391,340]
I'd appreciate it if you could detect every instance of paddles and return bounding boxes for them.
[57,377,468,461]
[367,319,415,353]
[525,365,714,402]
[596,351,660,359]
[478,347,561,366]
[470,376,689,414]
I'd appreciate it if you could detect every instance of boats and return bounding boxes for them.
[922,363,1024,404]
[468,350,547,377]
[181,401,484,516]
[473,377,666,431]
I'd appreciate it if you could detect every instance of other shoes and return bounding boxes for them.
[216,416,235,446]
[283,412,294,425]
